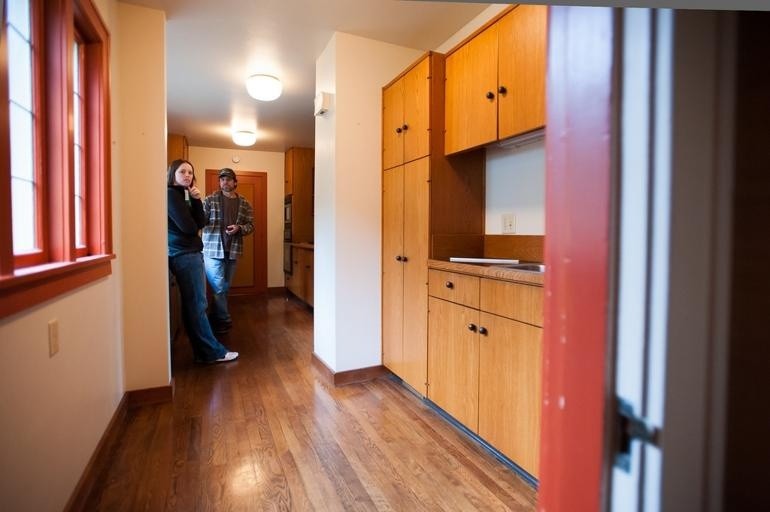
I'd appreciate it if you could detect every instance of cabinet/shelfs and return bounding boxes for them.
[284,148,294,196]
[381,51,484,398]
[291,242,314,308]
[444,4,547,162]
[426,261,544,482]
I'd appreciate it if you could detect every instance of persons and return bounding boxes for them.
[168,159,239,363]
[198,168,254,336]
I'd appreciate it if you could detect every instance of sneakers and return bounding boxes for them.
[193,352,239,364]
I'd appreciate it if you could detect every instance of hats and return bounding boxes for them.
[219,168,235,179]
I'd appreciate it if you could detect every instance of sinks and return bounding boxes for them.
[489,263,546,276]
